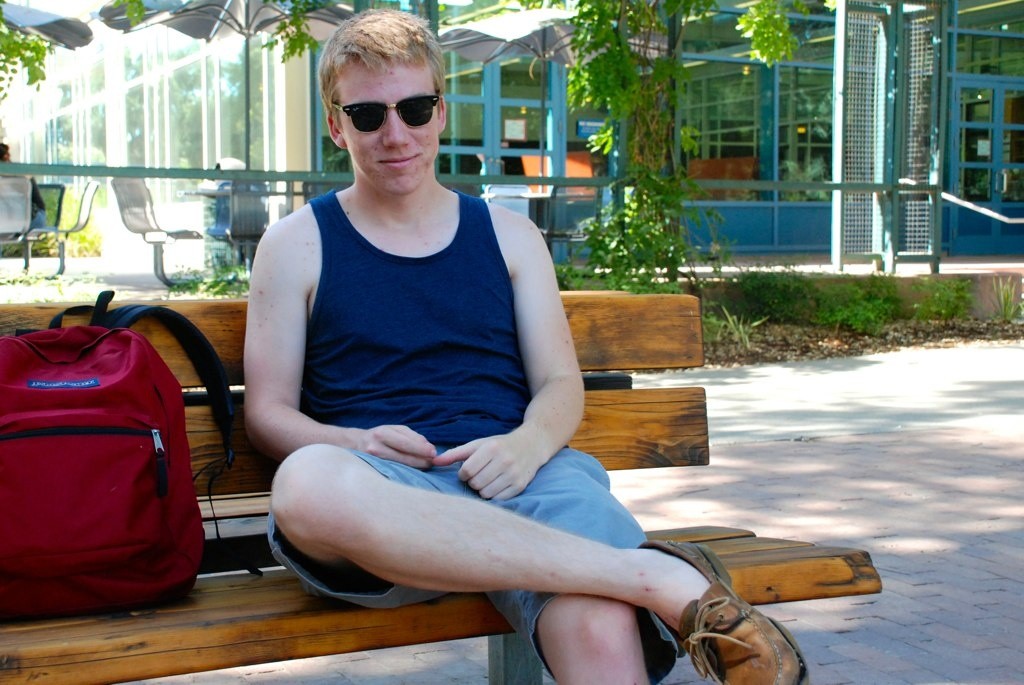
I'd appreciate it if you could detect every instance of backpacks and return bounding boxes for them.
[0,289,235,623]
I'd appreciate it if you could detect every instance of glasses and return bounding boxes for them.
[333,94,440,133]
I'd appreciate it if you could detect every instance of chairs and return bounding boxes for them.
[0,174,603,287]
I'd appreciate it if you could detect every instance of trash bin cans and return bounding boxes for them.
[200,157,247,266]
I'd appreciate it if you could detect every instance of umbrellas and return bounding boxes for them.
[435,7,666,194]
[0,1,95,52]
[99,0,362,193]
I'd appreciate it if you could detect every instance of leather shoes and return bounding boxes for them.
[638,538,810,685]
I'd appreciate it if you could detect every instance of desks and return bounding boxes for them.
[482,193,601,231]
[180,189,312,282]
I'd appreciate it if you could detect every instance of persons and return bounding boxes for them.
[0,142,47,233]
[240,9,809,685]
[474,142,510,192]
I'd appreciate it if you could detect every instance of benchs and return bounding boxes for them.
[0,292,883,685]
